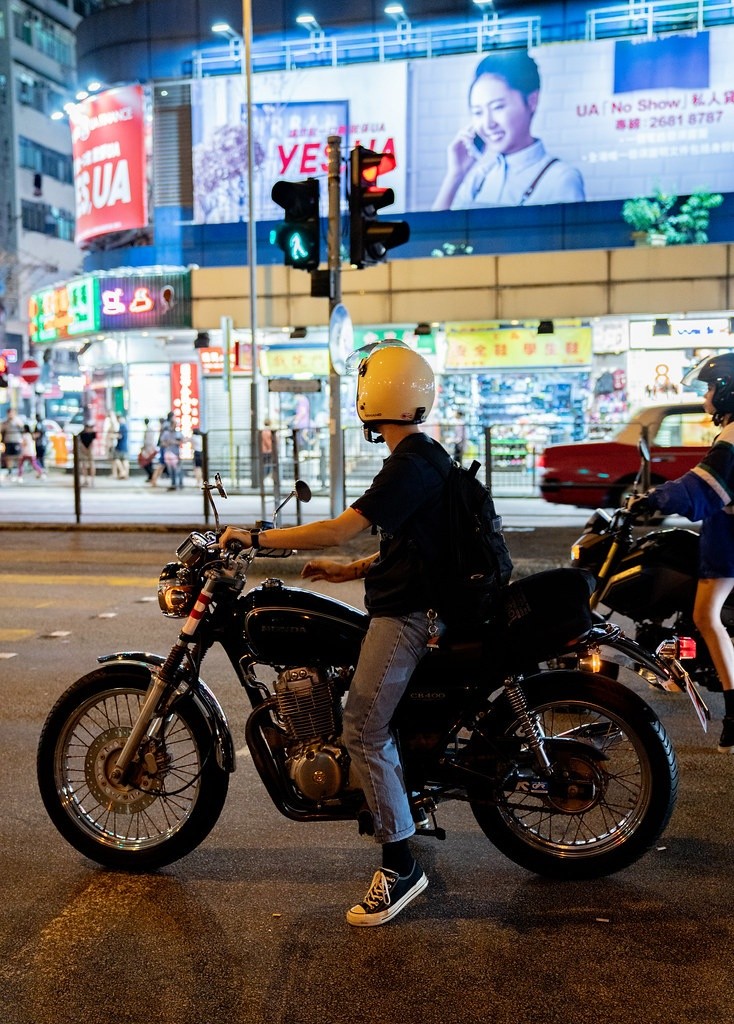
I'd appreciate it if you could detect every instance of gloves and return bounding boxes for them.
[628,493,657,518]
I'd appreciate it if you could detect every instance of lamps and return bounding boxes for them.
[290,327,306,338]
[87,78,113,92]
[193,333,209,348]
[295,14,323,39]
[384,3,411,31]
[537,321,553,334]
[414,323,431,335]
[652,318,671,336]
[211,22,242,46]
[472,0,499,20]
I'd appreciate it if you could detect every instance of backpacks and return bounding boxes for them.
[383,449,513,591]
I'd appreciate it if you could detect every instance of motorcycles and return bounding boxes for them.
[35,474,680,884]
[561,422,733,706]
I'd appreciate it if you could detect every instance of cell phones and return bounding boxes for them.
[469,135,487,155]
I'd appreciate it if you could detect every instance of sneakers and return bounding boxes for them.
[346,859,429,927]
[411,808,429,826]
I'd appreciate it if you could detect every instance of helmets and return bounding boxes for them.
[680,353,734,426]
[345,338,435,425]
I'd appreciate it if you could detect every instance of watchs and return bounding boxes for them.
[248,528,262,550]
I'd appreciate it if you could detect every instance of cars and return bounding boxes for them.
[2,410,101,472]
[536,403,722,513]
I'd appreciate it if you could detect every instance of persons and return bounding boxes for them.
[75,420,97,487]
[454,411,465,464]
[629,352,734,753]
[293,392,315,446]
[433,50,586,212]
[261,419,273,477]
[140,412,203,487]
[226,339,453,927]
[117,416,130,477]
[0,408,46,480]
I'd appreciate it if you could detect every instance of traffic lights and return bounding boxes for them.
[349,145,411,269]
[270,179,321,273]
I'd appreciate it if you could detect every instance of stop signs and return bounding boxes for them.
[19,360,40,384]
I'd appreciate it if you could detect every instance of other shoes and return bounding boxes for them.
[717,715,734,754]
